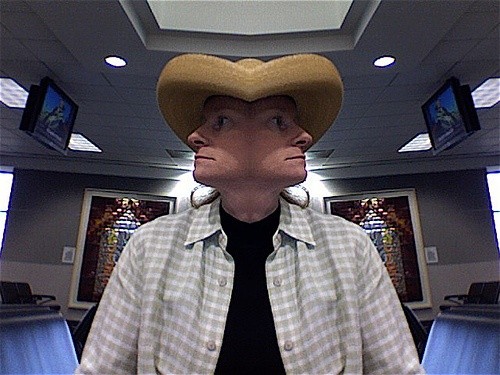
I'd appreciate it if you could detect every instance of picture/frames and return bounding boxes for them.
[424,246,440,265]
[68,188,176,311]
[323,188,434,311]
[61,245,76,266]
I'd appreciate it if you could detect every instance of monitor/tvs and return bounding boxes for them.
[421,76,483,157]
[18,77,80,157]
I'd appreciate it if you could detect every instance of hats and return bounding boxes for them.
[155,53,345,153]
